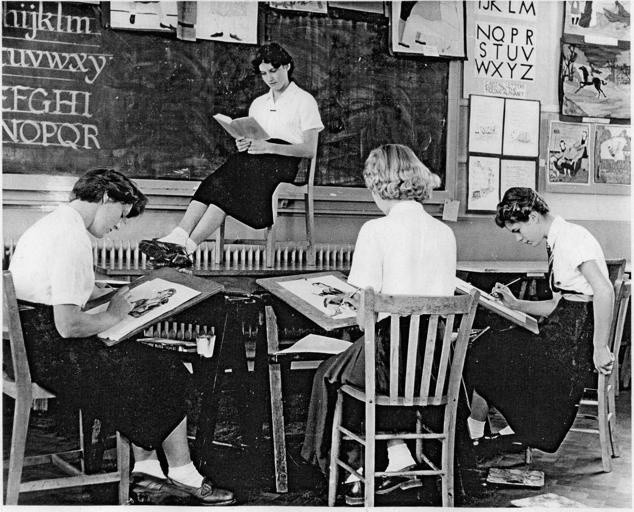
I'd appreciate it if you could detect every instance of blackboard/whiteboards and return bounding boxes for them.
[2,0,457,204]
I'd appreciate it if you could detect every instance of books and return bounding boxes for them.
[211,114,272,142]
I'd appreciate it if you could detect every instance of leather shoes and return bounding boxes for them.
[336,480,364,505]
[375,465,422,496]
[126,473,193,504]
[170,479,236,506]
[472,433,527,453]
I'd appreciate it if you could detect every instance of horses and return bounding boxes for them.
[573,65,608,99]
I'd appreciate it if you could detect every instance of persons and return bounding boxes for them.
[128,287,177,318]
[461,186,617,471]
[311,279,358,316]
[397,0,458,53]
[209,1,251,41]
[3,166,237,506]
[127,1,177,30]
[551,124,589,179]
[299,141,459,506]
[139,41,327,268]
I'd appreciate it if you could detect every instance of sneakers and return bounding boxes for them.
[138,240,193,268]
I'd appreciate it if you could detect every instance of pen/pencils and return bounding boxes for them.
[348,289,360,298]
[105,283,119,293]
[488,276,522,296]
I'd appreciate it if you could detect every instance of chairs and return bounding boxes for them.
[214,127,322,269]
[324,286,481,508]
[3,269,134,506]
[521,257,633,476]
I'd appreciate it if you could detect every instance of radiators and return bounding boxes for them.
[79,241,358,346]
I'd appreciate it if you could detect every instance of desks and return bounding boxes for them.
[100,261,545,497]
